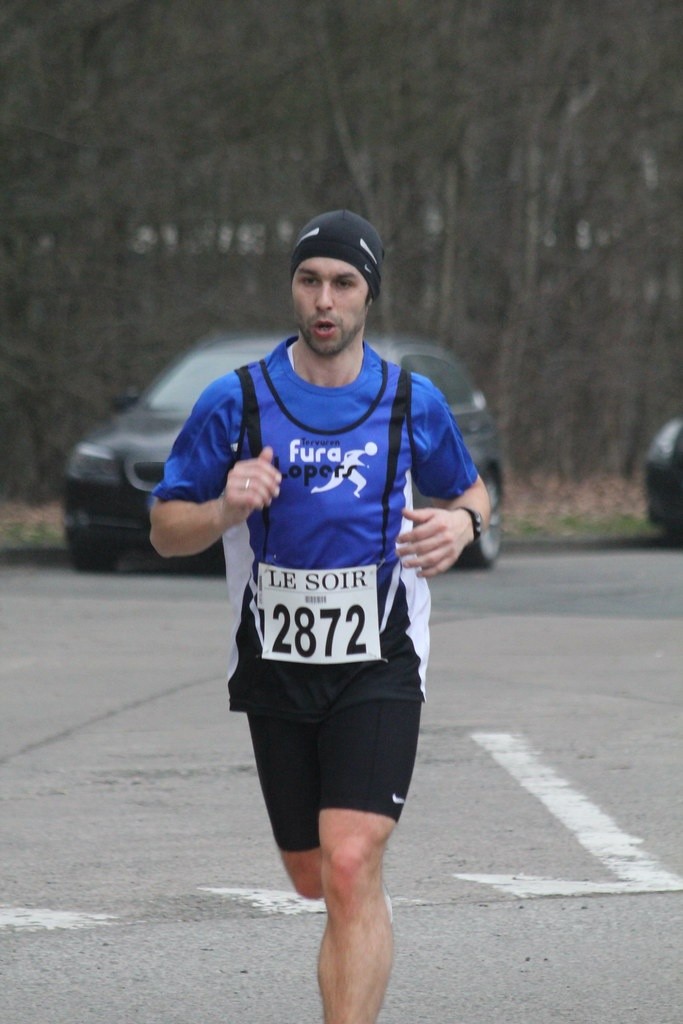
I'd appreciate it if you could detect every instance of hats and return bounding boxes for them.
[291,209,383,301]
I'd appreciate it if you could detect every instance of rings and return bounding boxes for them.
[245,478,249,490]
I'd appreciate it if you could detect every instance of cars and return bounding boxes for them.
[643,411,683,543]
[62,326,509,575]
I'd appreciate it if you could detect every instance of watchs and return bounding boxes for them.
[453,506,482,543]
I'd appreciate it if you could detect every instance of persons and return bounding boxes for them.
[149,210,492,1024]
